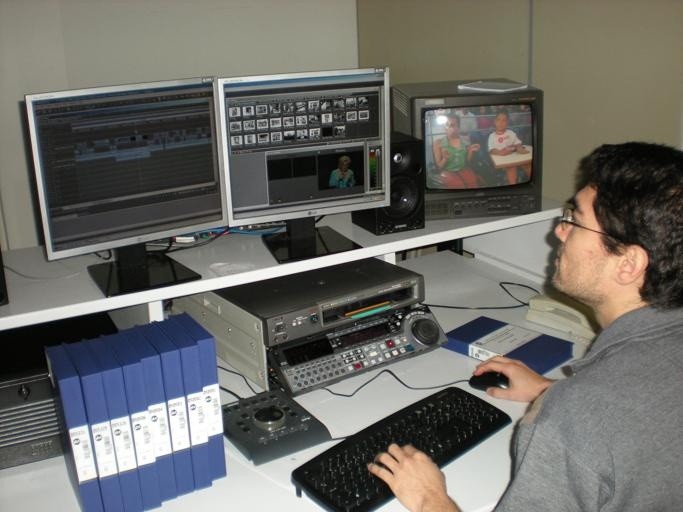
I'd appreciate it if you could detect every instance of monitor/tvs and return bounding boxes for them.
[22,77,228,296]
[391,77,543,220]
[218,67,390,263]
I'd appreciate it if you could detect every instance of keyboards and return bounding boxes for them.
[291,387,512,512]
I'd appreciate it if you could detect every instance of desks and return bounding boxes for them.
[0,196,591,512]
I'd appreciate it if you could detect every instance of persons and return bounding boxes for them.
[431,104,531,189]
[366,140,683,512]
[328,155,357,188]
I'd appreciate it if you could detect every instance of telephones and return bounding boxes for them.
[526,291,599,340]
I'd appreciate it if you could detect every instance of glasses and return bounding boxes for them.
[559,209,606,235]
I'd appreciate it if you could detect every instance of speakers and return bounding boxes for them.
[351,132,425,235]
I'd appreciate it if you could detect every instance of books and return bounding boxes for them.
[44,306,229,512]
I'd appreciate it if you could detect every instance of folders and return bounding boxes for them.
[43,310,226,512]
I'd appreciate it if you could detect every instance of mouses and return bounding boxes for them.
[469,372,508,390]
